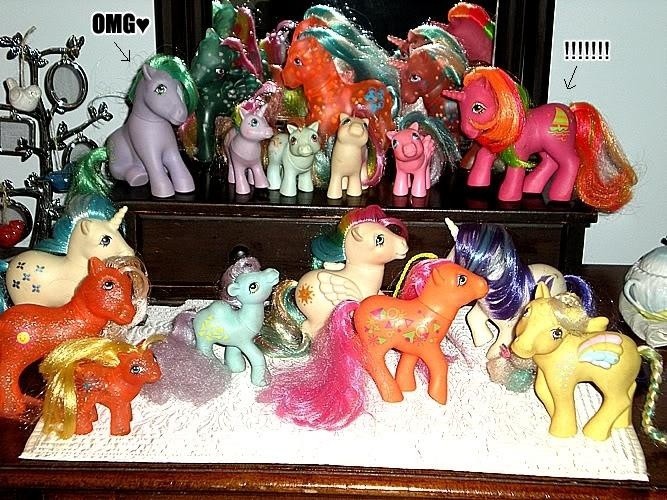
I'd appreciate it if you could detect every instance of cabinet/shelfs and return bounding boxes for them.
[0,177,667,500]
